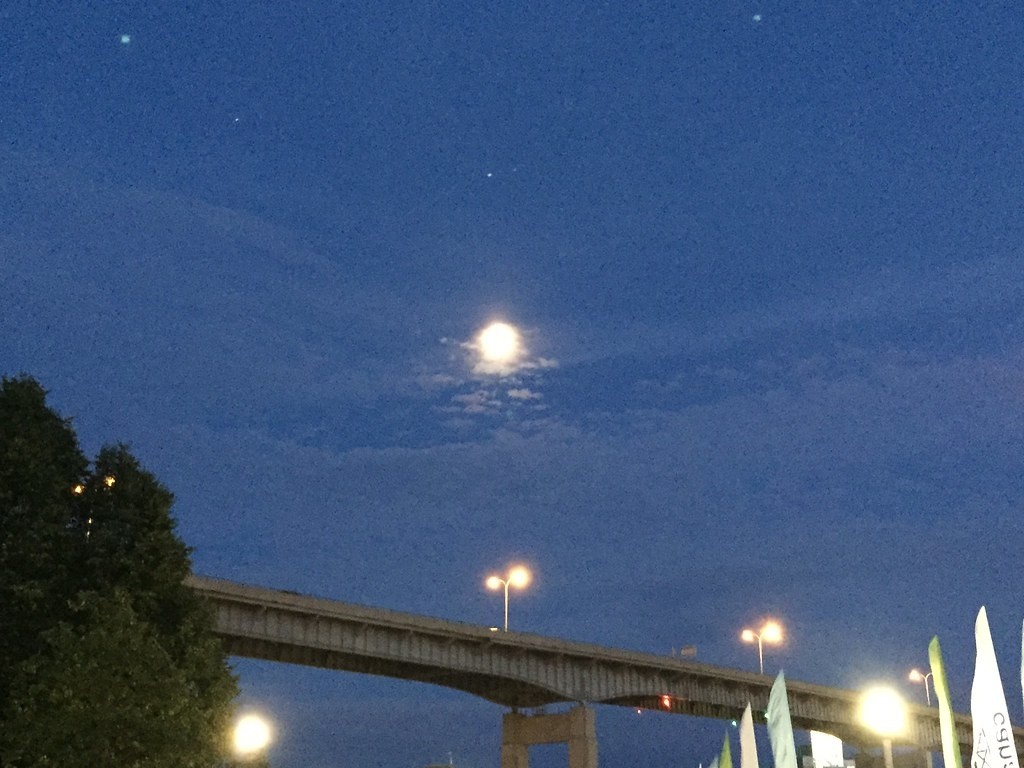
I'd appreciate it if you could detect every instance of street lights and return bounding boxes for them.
[738,618,782,676]
[905,669,934,706]
[854,685,909,768]
[485,566,530,630]
